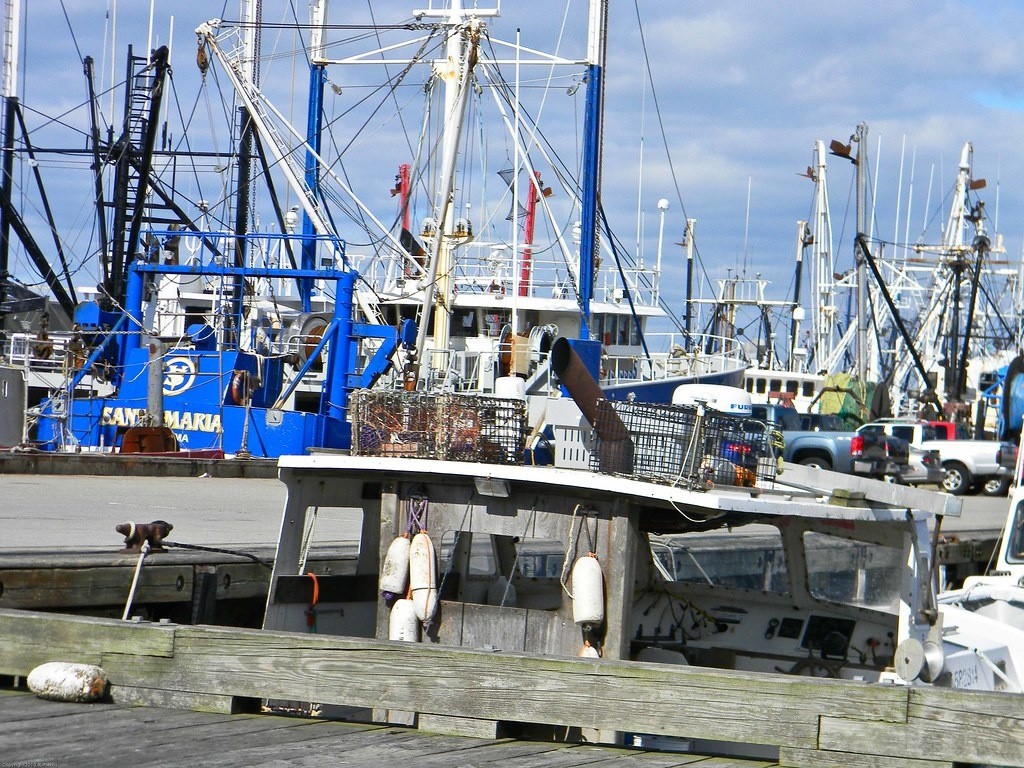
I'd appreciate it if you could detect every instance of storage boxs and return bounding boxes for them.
[588,393,784,486]
[346,388,527,461]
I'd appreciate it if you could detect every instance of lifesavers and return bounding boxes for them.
[232,370,253,406]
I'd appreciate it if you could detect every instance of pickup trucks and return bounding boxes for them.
[724,404,910,475]
[854,424,1019,498]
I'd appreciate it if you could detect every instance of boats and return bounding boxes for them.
[681,121,1024,432]
[0,1,748,468]
[258,338,1024,761]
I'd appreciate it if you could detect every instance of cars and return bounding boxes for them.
[720,437,760,468]
[883,445,947,485]
